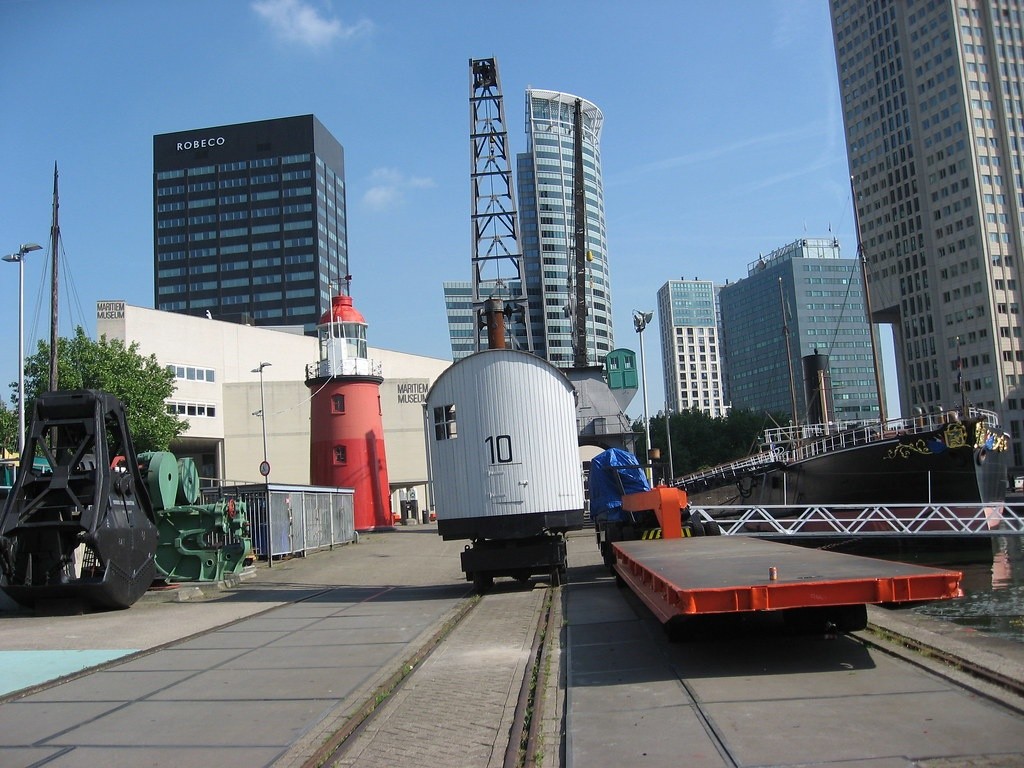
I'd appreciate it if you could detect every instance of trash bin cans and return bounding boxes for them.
[422,509,429,524]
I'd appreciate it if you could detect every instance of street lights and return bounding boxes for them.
[632,308,654,490]
[250,361,272,486]
[0,242,45,467]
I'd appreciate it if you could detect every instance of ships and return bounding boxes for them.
[669,274,1010,536]
